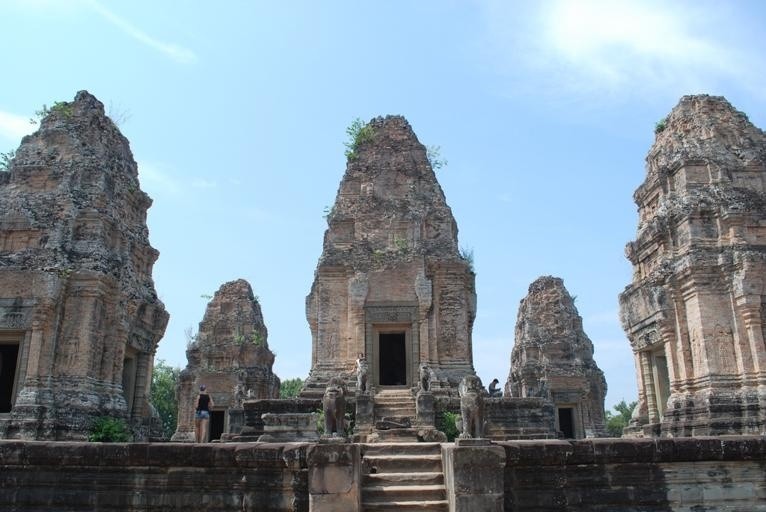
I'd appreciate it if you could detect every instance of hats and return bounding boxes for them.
[199,385,206,391]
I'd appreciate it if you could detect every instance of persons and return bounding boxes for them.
[195,385,214,443]
